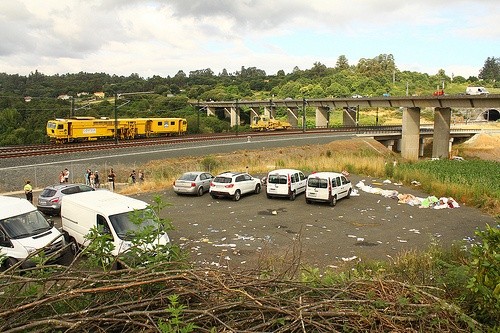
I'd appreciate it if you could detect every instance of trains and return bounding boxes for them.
[46,116,188,145]
[251,115,291,131]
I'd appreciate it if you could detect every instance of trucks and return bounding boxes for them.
[466,87,490,96]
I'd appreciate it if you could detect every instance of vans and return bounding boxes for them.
[304,171,353,206]
[60,189,169,273]
[0,195,67,275]
[265,169,308,200]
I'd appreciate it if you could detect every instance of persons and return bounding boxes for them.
[24,181,33,204]
[139,170,144,184]
[84,169,98,188]
[60,168,69,183]
[108,169,116,191]
[130,170,137,184]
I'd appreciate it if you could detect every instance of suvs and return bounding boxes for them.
[38,182,98,215]
[209,171,262,200]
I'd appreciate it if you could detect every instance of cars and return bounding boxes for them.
[173,171,214,196]
